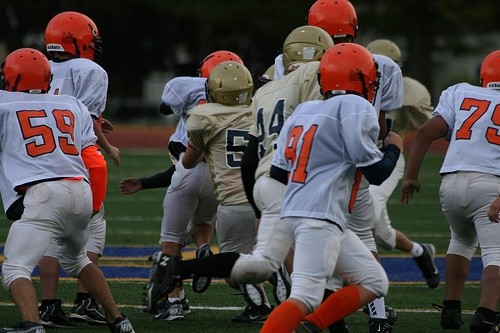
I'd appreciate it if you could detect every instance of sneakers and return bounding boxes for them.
[143,294,192,313]
[413,244,441,290]
[432,299,500,333]
[39,302,78,328]
[155,299,185,322]
[231,303,275,323]
[69,298,109,325]
[105,312,135,333]
[3,320,47,333]
[193,244,214,293]
[148,250,183,310]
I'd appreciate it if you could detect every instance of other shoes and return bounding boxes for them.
[327,318,350,333]
[295,319,323,333]
[364,304,397,333]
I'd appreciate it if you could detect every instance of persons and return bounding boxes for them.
[0,48,136,333]
[37,11,108,329]
[399,50,500,333]
[119,0,439,333]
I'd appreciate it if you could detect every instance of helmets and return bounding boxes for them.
[480,50,500,89]
[317,43,381,106]
[1,48,51,93]
[307,0,358,45]
[366,40,401,64]
[283,26,334,75]
[44,12,102,63]
[199,50,275,107]
